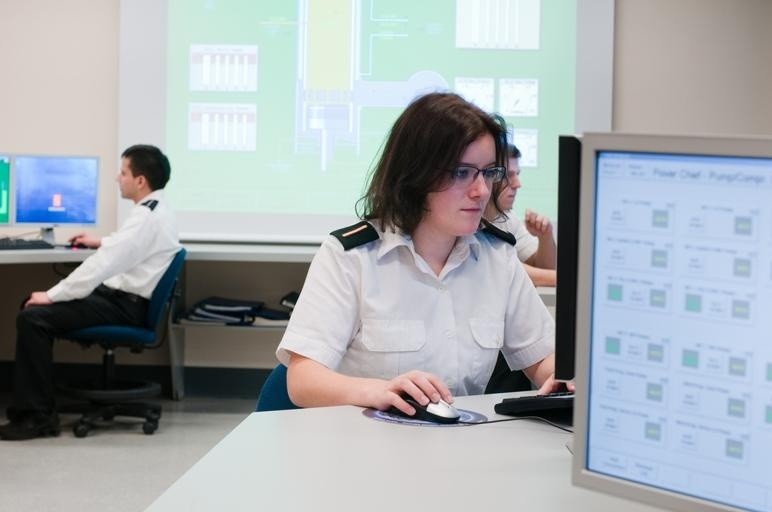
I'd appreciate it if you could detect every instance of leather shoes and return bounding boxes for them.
[0,404,62,441]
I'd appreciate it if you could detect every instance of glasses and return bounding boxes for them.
[450,165,506,186]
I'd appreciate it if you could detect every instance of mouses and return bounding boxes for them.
[64,241,88,250]
[389,390,460,423]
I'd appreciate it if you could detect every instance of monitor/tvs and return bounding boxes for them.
[571,129,771,510]
[15,154,100,247]
[554,134,582,385]
[0,152,12,226]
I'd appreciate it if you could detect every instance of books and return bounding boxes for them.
[177,289,300,326]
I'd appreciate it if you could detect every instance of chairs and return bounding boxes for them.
[54,248,187,437]
[255,363,302,411]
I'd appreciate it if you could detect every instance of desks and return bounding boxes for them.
[144,390,683,512]
[1,244,557,400]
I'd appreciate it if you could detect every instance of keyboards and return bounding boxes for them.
[495,392,574,425]
[0,239,55,249]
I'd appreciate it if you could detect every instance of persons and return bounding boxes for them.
[483,144,557,287]
[275,91,574,417]
[0,146,181,440]
